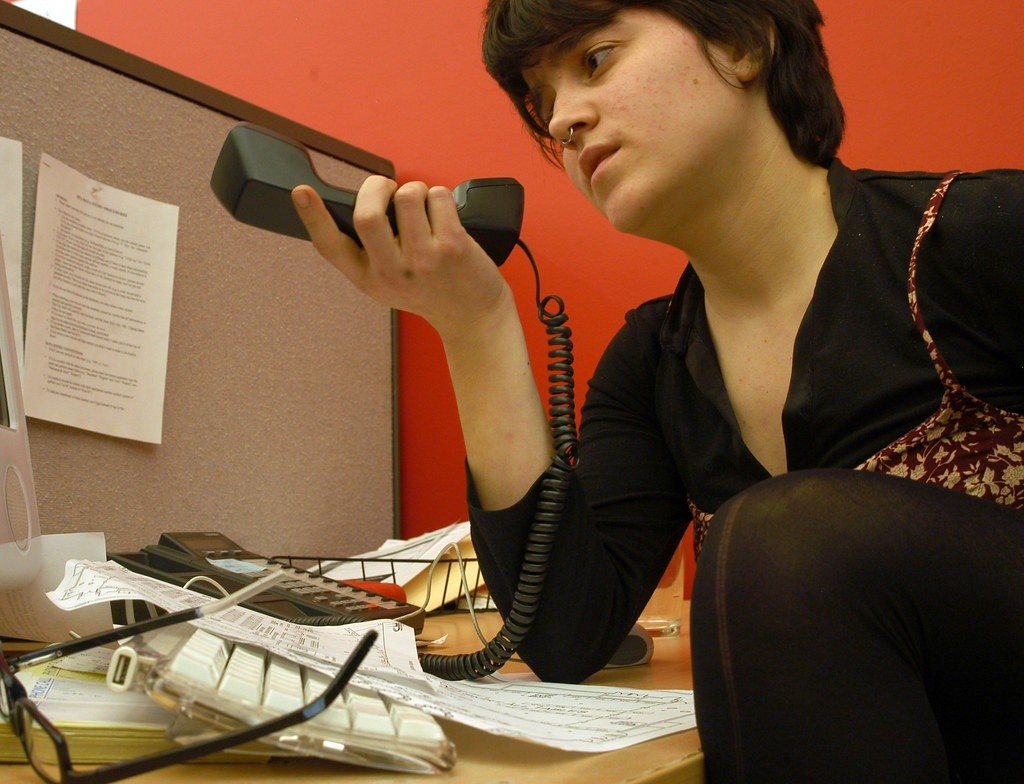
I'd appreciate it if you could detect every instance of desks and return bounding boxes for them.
[0,569,707,784]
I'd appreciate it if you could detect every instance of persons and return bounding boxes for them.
[288,0,1024,783]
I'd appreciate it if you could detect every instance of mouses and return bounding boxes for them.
[605,622,654,668]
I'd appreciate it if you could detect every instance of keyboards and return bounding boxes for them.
[107,616,457,776]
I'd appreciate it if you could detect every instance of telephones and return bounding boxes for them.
[210,124,524,269]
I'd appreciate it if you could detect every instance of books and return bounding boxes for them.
[0,642,272,766]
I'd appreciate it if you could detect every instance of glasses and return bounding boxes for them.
[0,570,378,784]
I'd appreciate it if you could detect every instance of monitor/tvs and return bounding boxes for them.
[0,244,40,548]
